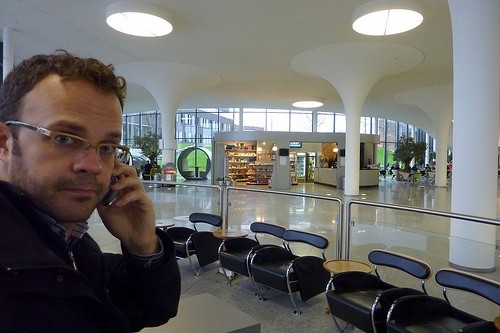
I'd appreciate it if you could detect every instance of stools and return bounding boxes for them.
[200,171,206,177]
[183,171,195,177]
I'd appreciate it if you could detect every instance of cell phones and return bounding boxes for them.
[100,175,120,207]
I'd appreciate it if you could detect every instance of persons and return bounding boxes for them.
[0,49,180,333]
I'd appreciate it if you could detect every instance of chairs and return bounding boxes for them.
[162,213,500,333]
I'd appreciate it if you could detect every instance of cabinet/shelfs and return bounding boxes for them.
[226,147,317,185]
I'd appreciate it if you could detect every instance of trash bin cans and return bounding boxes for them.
[153,172,161,188]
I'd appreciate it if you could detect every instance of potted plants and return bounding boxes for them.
[134,134,163,180]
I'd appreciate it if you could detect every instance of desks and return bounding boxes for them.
[323,259,373,289]
[155,220,175,229]
[139,292,261,333]
[213,231,248,250]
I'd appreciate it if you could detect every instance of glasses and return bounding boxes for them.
[6,120,130,168]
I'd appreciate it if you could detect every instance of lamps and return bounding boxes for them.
[351,0,424,36]
[106,2,175,38]
[290,96,325,109]
